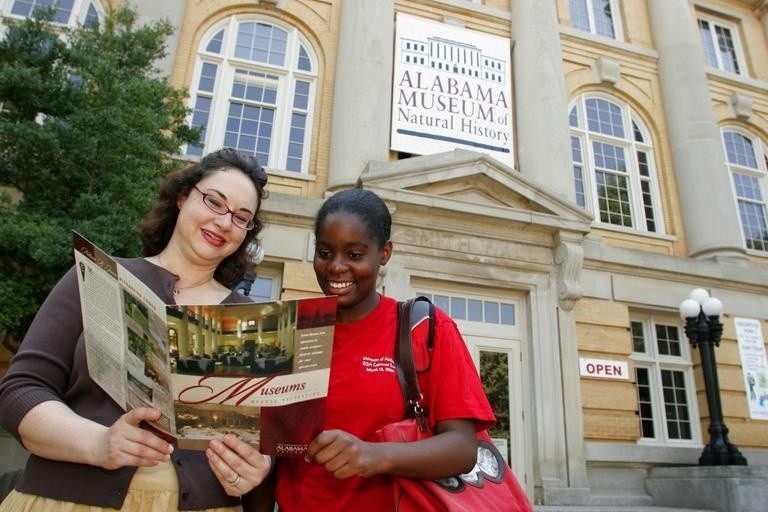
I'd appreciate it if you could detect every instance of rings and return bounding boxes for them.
[230,476,240,485]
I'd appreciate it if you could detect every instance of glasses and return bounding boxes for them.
[194,185,255,231]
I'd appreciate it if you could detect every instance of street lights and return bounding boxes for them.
[680,289,747,466]
[235,240,264,296]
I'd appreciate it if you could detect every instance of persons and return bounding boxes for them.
[265,176,497,512]
[0,147,274,512]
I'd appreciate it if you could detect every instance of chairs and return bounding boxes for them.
[174,351,293,374]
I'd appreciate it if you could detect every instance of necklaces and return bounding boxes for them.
[156,253,213,296]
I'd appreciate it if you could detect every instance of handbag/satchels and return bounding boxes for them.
[374,297,534,512]
